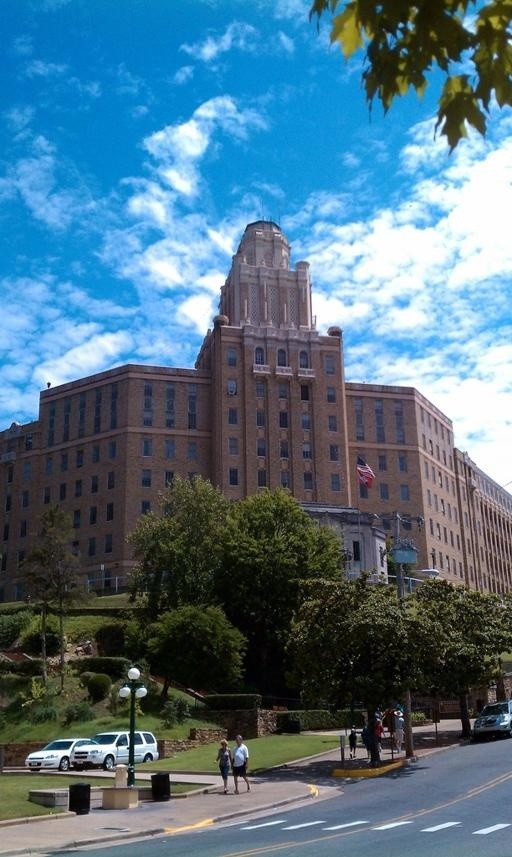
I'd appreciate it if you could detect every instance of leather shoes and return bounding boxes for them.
[224,788,227,793]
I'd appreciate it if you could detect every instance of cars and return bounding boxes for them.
[26,737,98,771]
[475,700,512,740]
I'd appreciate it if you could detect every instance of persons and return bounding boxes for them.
[232,735,251,793]
[349,728,357,759]
[215,739,232,794]
[362,720,371,759]
[374,701,406,753]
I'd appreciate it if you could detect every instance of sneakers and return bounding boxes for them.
[234,790,239,794]
[247,785,251,793]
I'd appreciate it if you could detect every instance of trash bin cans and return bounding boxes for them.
[151,772,170,801]
[69,783,90,815]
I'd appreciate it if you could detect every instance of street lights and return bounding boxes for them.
[119,665,148,785]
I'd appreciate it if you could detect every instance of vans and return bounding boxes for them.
[71,730,159,769]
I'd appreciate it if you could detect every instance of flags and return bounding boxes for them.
[356,455,376,486]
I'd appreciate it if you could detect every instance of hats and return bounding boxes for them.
[394,710,403,717]
